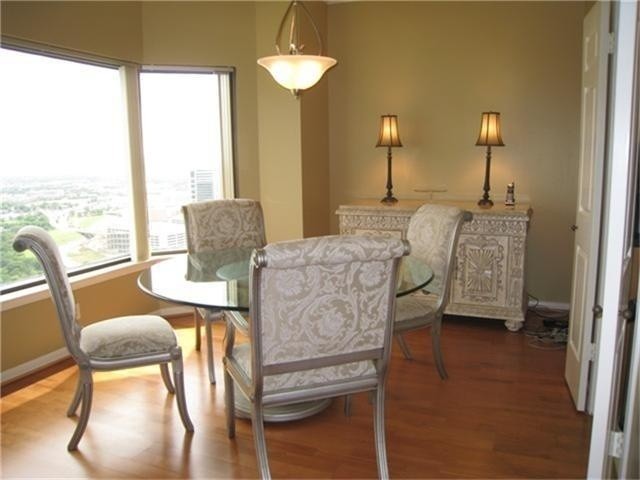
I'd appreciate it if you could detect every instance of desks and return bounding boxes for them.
[135,240,441,425]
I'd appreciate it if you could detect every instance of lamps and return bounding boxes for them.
[258,0,337,97]
[375,114,403,204]
[474,111,505,209]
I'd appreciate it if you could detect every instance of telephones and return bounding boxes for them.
[505,182,516,206]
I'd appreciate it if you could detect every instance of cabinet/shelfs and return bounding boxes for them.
[333,192,532,333]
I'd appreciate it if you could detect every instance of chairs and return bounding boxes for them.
[366,198,472,406]
[12,223,197,455]
[219,233,415,476]
[178,199,290,386]
